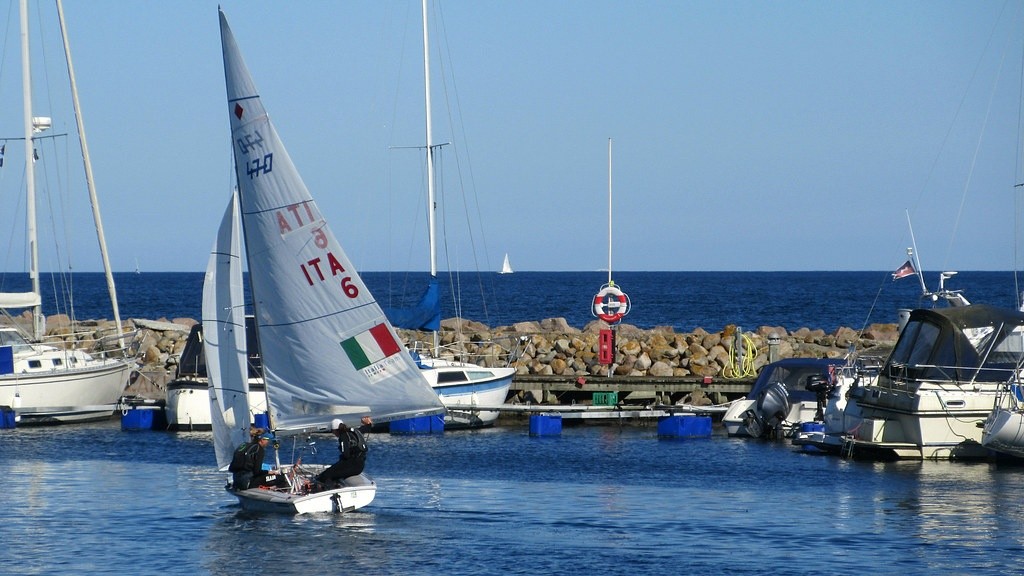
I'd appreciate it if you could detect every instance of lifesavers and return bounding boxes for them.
[594,286,628,323]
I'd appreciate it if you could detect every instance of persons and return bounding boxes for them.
[228,432,288,491]
[308,418,367,491]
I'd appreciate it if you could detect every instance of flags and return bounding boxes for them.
[891,261,915,281]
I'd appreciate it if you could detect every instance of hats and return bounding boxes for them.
[328,419,342,430]
[253,431,274,439]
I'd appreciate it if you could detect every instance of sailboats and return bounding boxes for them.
[0,0,148,425]
[498,252,514,275]
[721,1,1024,464]
[164,1,533,516]
